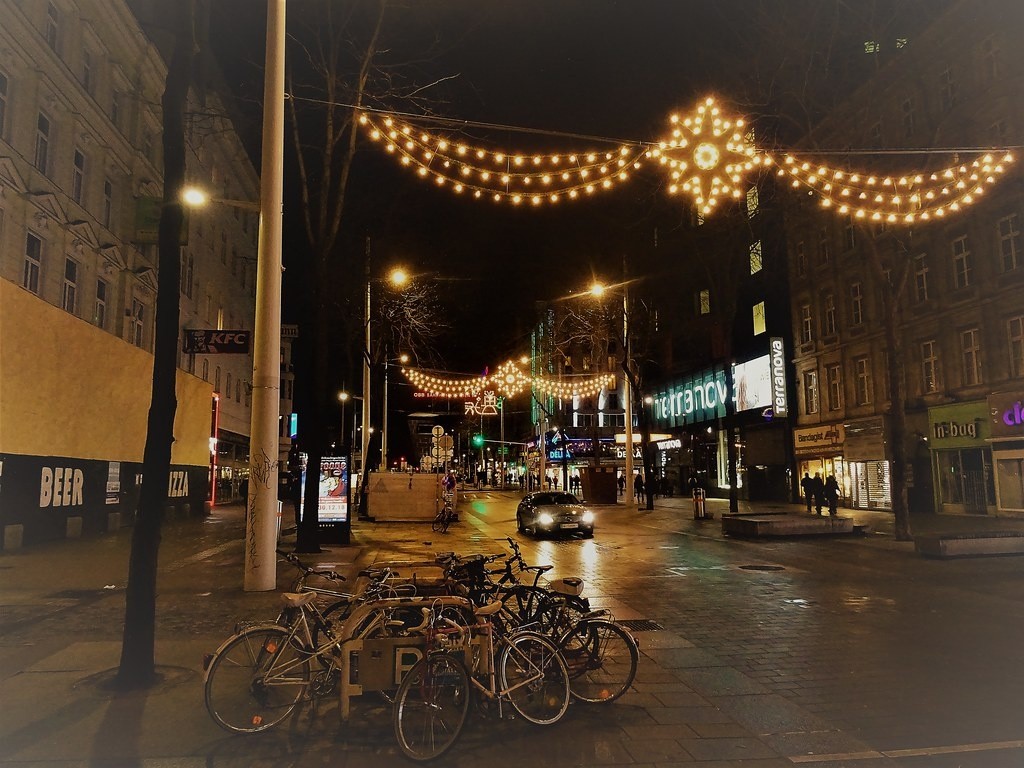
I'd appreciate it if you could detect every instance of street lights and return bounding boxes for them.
[339,236,407,482]
[370,344,410,471]
[175,183,283,592]
[590,255,652,509]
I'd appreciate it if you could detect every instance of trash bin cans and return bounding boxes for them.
[692,488,705,518]
[276,501,283,544]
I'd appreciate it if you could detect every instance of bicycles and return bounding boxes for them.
[432,496,455,533]
[203,537,641,765]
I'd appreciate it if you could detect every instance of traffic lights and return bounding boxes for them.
[475,438,482,443]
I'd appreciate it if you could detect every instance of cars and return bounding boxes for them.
[516,491,594,539]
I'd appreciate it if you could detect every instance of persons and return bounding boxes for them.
[553,476,558,489]
[812,472,825,515]
[507,473,524,486]
[574,476,581,495]
[801,473,814,512]
[634,474,644,503]
[618,474,626,496]
[824,475,841,515]
[661,474,669,498]
[442,470,457,520]
[570,476,574,493]
[653,475,661,499]
